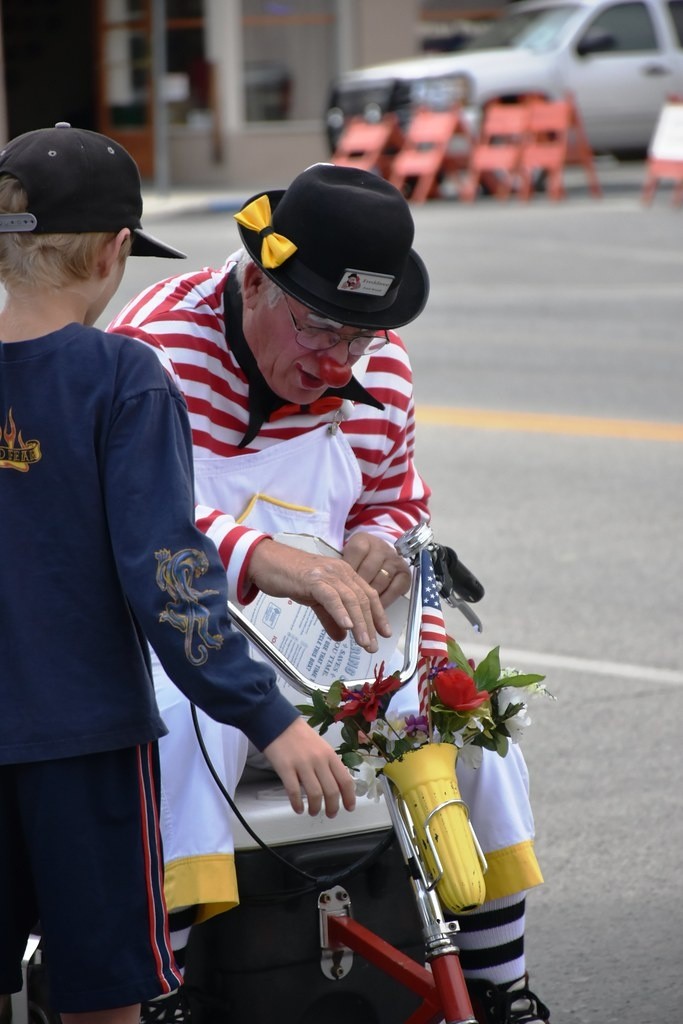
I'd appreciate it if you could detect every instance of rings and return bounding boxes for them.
[380,568,393,582]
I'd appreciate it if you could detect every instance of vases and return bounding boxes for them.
[383,741,487,916]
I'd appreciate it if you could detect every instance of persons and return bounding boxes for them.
[0,117,358,1018]
[105,163,550,1024]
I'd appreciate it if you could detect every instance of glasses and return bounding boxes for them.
[280,286,390,356]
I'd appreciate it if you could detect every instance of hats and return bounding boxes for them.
[233,165,429,330]
[0,122,186,259]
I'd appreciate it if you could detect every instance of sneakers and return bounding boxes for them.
[463,971,552,1024]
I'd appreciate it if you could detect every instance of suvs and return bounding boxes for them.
[326,0,682,192]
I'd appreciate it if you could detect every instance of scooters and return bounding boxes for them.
[24,516,493,1022]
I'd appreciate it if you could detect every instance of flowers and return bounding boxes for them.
[293,635,557,804]
